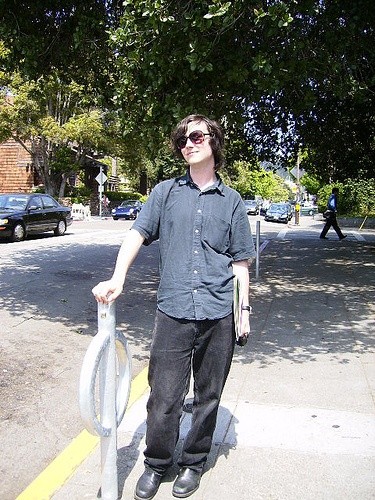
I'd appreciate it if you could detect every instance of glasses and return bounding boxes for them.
[176,130,210,149]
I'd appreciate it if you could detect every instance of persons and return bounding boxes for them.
[92,114,258,500]
[320,188,348,241]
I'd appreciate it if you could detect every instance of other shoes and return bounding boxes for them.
[340,235,347,240]
[320,237,328,240]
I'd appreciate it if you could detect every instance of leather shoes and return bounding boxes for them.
[172,468,202,496]
[134,469,163,500]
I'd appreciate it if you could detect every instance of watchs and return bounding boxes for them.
[242,305,252,313]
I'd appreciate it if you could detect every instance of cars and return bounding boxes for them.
[257,200,318,224]
[0,192,73,242]
[244,200,259,215]
[110,200,144,220]
